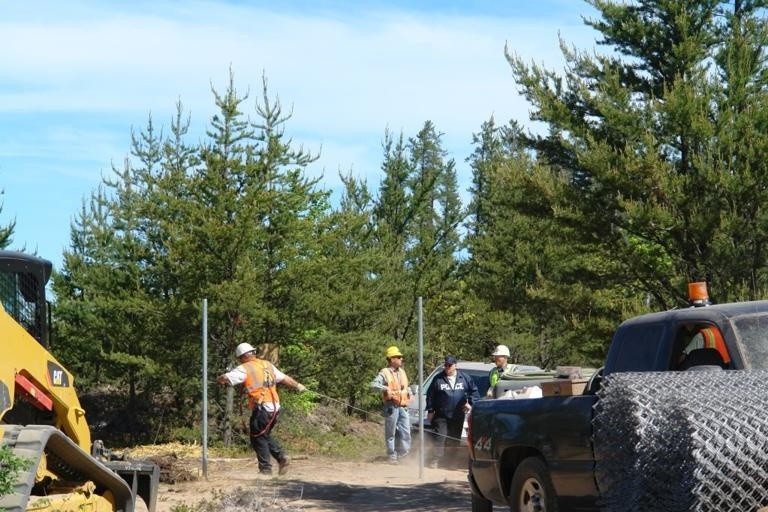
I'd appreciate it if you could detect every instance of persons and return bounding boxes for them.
[369,345,413,464]
[484,344,524,401]
[424,355,480,469]
[214,342,309,475]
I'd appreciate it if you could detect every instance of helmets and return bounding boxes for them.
[492,344,511,358]
[385,346,403,358]
[235,342,257,357]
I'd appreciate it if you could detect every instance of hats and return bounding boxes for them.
[442,356,457,365]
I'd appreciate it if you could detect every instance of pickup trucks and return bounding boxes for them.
[460,281,768,511]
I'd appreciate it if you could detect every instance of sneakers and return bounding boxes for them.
[279,455,290,475]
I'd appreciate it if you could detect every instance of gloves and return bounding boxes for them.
[296,383,305,393]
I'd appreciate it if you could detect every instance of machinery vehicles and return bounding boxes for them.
[0,251,161,512]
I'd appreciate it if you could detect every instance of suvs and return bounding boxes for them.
[407,362,541,454]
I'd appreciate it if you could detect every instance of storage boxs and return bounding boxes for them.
[540,377,589,397]
